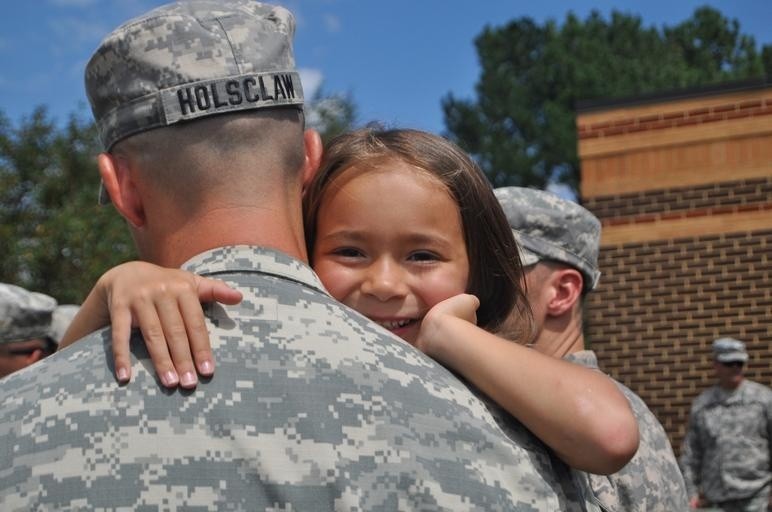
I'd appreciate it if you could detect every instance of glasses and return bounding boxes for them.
[724,362,744,368]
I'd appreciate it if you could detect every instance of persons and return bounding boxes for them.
[1,283,57,379]
[57,129,640,476]
[678,338,772,512]
[492,186,690,512]
[1,0,583,512]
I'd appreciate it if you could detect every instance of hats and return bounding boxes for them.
[84,1,306,206]
[0,283,60,350]
[713,337,750,364]
[491,184,603,291]
